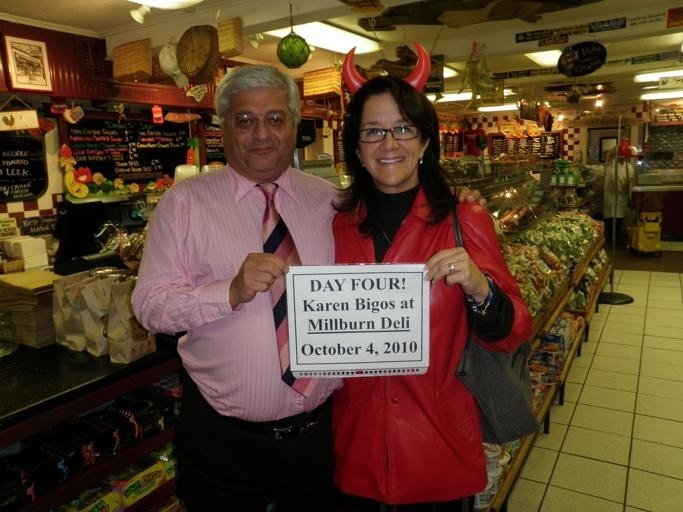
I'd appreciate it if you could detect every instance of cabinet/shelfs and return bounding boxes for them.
[0,332,191,512]
[418,173,615,509]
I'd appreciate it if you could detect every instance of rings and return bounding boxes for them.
[447,263,457,274]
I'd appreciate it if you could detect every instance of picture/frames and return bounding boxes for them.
[2,32,53,93]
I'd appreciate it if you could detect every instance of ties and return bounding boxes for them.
[255,182,317,400]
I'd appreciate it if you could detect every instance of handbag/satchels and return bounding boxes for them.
[451,199,537,445]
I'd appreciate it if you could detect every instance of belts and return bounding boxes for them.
[207,417,323,443]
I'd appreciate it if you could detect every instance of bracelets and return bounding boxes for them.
[461,289,493,316]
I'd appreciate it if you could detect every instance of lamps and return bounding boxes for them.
[130,5,151,25]
[248,33,264,49]
[277,4,310,69]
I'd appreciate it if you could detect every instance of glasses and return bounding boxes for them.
[357,125,419,143]
[229,112,291,131]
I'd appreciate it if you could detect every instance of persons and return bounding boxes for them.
[131,63,486,512]
[603,144,635,251]
[331,76,534,512]
